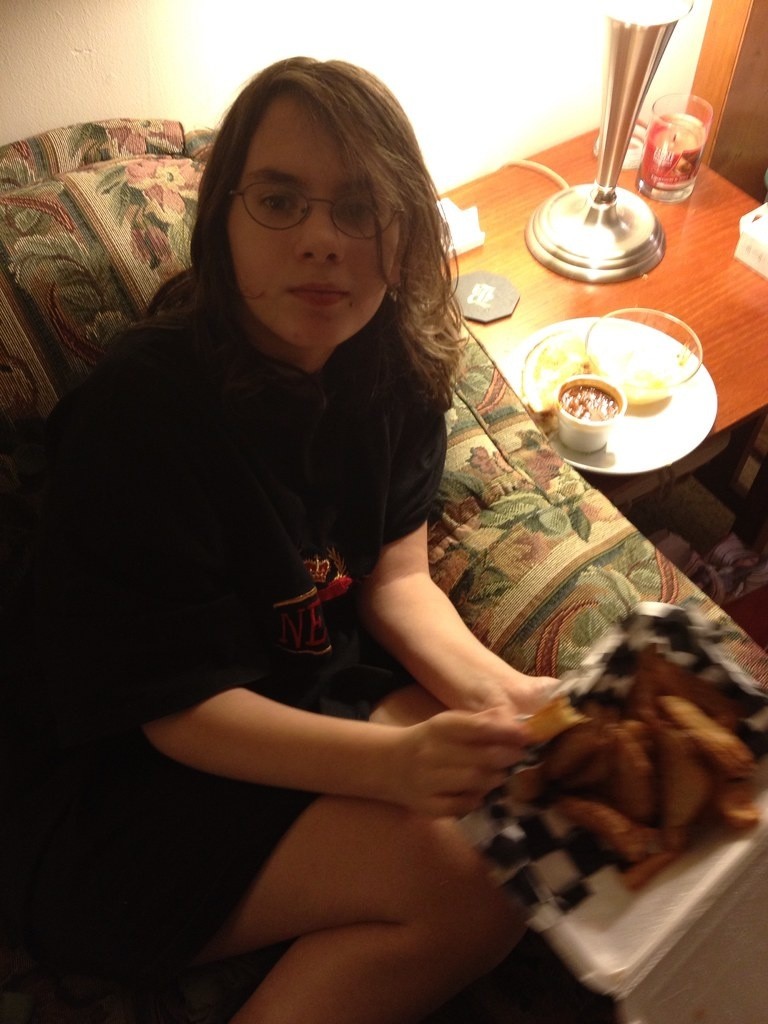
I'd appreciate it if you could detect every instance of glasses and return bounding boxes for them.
[227,184,403,238]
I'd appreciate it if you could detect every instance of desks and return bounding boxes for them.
[437,119,768,553]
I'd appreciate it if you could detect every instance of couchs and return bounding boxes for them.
[0,114,768,1024]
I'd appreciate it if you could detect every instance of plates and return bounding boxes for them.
[503,315,717,475]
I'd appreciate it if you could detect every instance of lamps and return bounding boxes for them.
[524,0,695,286]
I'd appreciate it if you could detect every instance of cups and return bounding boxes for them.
[634,92,713,204]
[556,374,628,452]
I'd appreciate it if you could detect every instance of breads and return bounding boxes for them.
[521,650,763,886]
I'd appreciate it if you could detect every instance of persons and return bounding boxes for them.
[0,57,564,1024]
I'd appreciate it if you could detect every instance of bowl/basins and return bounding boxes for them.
[587,308,703,406]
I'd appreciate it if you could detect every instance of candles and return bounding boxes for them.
[634,92,714,204]
